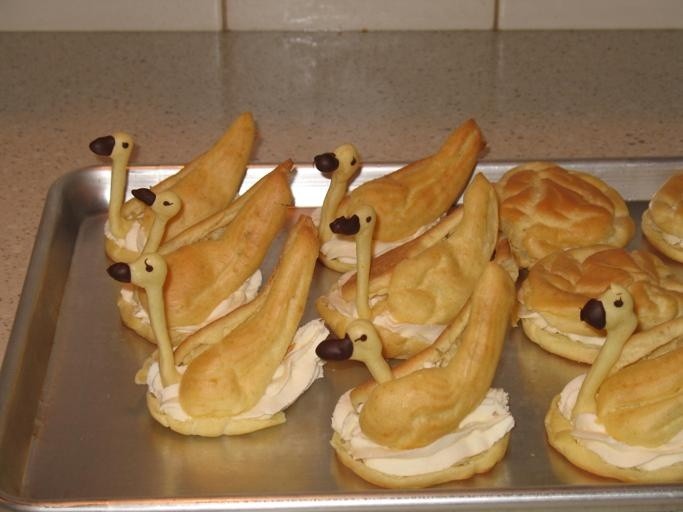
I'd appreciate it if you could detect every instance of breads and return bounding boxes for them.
[315,172,520,359]
[105,213,330,438]
[312,118,488,273]
[116,159,295,347]
[316,237,517,489]
[641,171,682,262]
[90,111,256,263]
[496,158,637,270]
[543,282,683,485]
[516,244,683,365]
